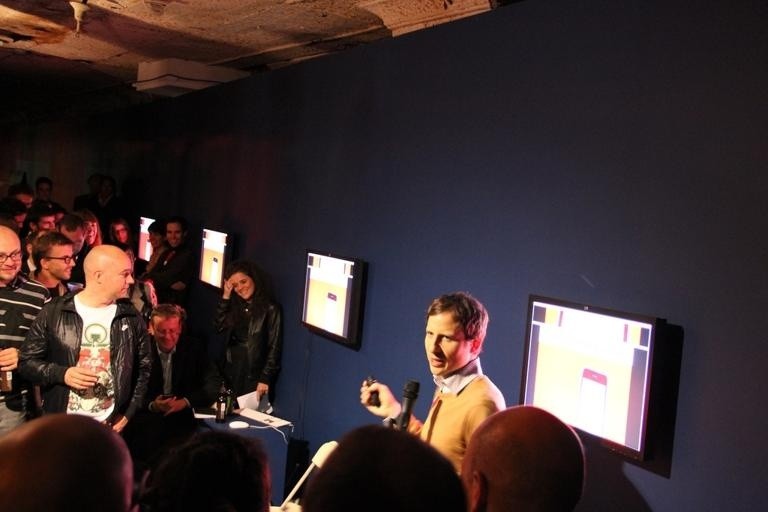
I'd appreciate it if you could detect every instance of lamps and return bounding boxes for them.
[271,439,339,512]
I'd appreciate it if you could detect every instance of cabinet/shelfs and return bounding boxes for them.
[225,386,235,414]
[214,381,227,423]
[0,370,12,392]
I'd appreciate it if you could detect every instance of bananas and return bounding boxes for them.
[239,407,292,428]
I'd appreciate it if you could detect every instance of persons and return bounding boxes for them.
[360,292,506,468]
[110,215,209,470]
[140,428,269,510]
[303,425,469,511]
[461,406,587,511]
[0,414,133,511]
[17,245,154,432]
[1,176,104,435]
[92,178,126,242]
[207,263,284,408]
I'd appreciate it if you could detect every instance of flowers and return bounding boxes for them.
[395,377,420,434]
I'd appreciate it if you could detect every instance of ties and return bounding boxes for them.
[197,227,232,294]
[518,293,667,467]
[298,248,368,352]
[136,214,157,264]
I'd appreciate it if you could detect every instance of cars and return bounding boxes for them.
[67,282,85,293]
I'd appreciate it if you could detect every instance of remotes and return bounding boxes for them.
[229,421,249,429]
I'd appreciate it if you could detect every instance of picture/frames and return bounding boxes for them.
[199,409,294,508]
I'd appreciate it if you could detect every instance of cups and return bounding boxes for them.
[0,250,23,263]
[40,253,75,264]
